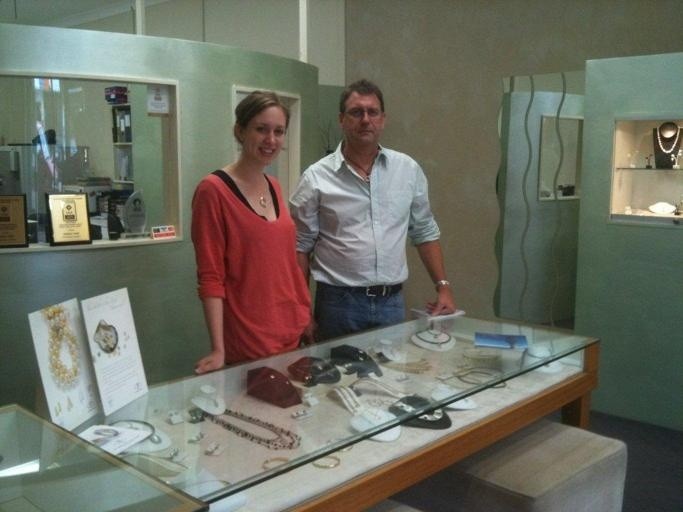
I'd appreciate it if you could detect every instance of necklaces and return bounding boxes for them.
[253,184,268,209]
[109,328,556,469]
[655,122,679,155]
[344,153,370,177]
[40,307,89,386]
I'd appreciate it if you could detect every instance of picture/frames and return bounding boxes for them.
[0,193,29,248]
[45,192,92,247]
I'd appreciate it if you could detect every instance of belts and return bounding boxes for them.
[337,284,401,297]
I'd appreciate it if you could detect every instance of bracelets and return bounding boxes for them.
[432,278,451,294]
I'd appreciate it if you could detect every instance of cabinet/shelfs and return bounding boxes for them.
[111,104,134,185]
[605,112,683,231]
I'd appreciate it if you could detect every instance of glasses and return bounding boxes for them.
[345,107,380,116]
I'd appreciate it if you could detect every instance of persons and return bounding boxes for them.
[288,79,456,343]
[190,94,316,377]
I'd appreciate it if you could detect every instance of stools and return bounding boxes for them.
[363,498,422,511]
[387,418,629,511]
